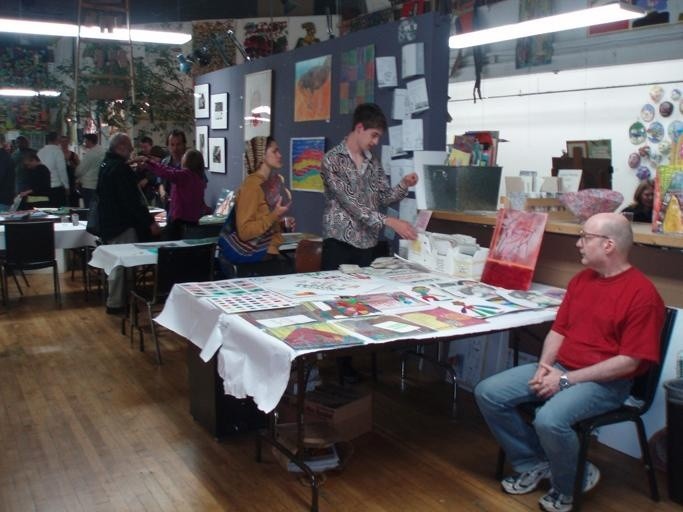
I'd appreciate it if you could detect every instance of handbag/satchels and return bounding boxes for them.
[218,204,273,265]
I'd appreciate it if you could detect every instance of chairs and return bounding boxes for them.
[130,242,217,364]
[1,221,59,306]
[498,296,676,512]
[293,238,329,276]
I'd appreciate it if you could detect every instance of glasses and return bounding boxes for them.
[579,229,609,239]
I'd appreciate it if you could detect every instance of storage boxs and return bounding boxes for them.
[424,163,502,212]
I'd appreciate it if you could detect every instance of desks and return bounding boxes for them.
[154,263,569,512]
[88,233,322,350]
[0,219,97,300]
[158,214,226,236]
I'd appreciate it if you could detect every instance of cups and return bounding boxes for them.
[70,213,79,226]
[280,217,292,232]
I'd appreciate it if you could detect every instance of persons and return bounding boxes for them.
[319,102,419,272]
[473,210,665,511]
[0,130,215,283]
[615,179,654,221]
[86,131,160,315]
[216,135,296,281]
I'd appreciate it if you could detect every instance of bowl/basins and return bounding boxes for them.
[561,188,624,221]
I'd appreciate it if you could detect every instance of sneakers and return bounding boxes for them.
[500,461,552,495]
[538,461,601,512]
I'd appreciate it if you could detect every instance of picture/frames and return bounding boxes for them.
[192,65,274,173]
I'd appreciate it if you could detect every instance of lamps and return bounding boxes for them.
[448,0,643,52]
[0,0,192,46]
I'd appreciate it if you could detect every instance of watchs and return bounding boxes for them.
[558,373,570,392]
